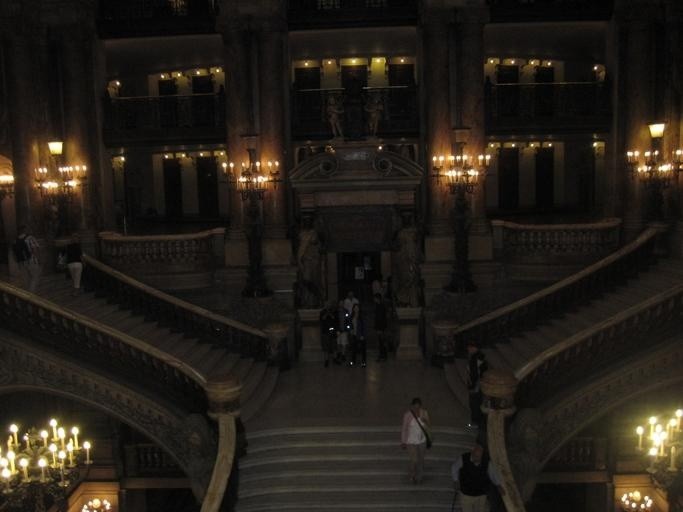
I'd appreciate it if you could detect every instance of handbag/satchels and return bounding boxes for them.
[427,440,431,448]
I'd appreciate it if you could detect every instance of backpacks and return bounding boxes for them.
[13,234,31,262]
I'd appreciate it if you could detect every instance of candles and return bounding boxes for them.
[624,147,683,178]
[0,421,91,484]
[431,154,493,187]
[34,166,90,196]
[634,412,682,478]
[221,161,281,193]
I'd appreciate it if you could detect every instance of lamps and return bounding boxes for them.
[47,136,65,165]
[647,120,668,145]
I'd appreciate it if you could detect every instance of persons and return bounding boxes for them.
[364,96,380,136]
[66,233,84,296]
[478,353,488,377]
[466,360,477,390]
[450,445,490,512]
[320,274,393,368]
[326,95,345,138]
[400,397,433,485]
[12,224,42,293]
